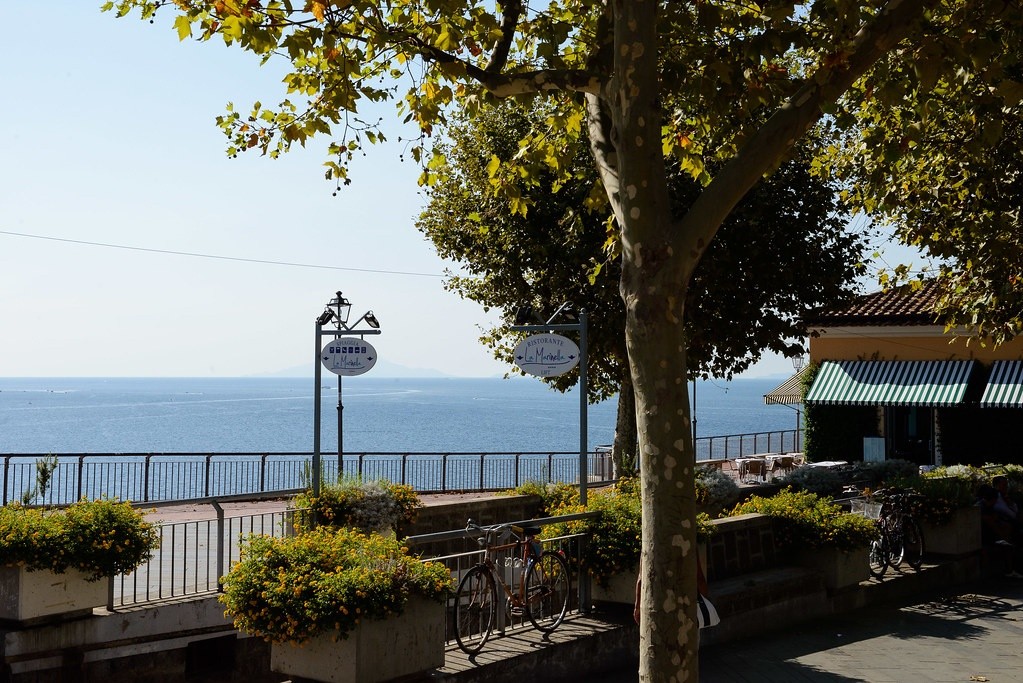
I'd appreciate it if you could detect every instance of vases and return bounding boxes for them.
[904,507,984,556]
[590,561,640,606]
[268,590,445,683]
[780,545,873,591]
[0,559,113,624]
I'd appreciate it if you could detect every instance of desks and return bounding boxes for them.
[736,451,804,470]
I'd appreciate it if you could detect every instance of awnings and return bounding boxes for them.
[980,360,1023,408]
[805,358,976,407]
[766,363,810,404]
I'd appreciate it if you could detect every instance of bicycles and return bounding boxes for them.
[843,485,924,577]
[453,520,570,653]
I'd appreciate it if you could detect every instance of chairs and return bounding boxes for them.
[725,456,804,485]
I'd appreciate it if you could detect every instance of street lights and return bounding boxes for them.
[791,354,804,450]
[338,309,379,484]
[313,312,334,497]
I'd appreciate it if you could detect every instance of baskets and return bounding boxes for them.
[851,500,883,521]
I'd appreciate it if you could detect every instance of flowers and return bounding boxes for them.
[273,458,428,536]
[497,477,882,594]
[0,495,165,584]
[216,522,462,649]
[891,464,991,528]
